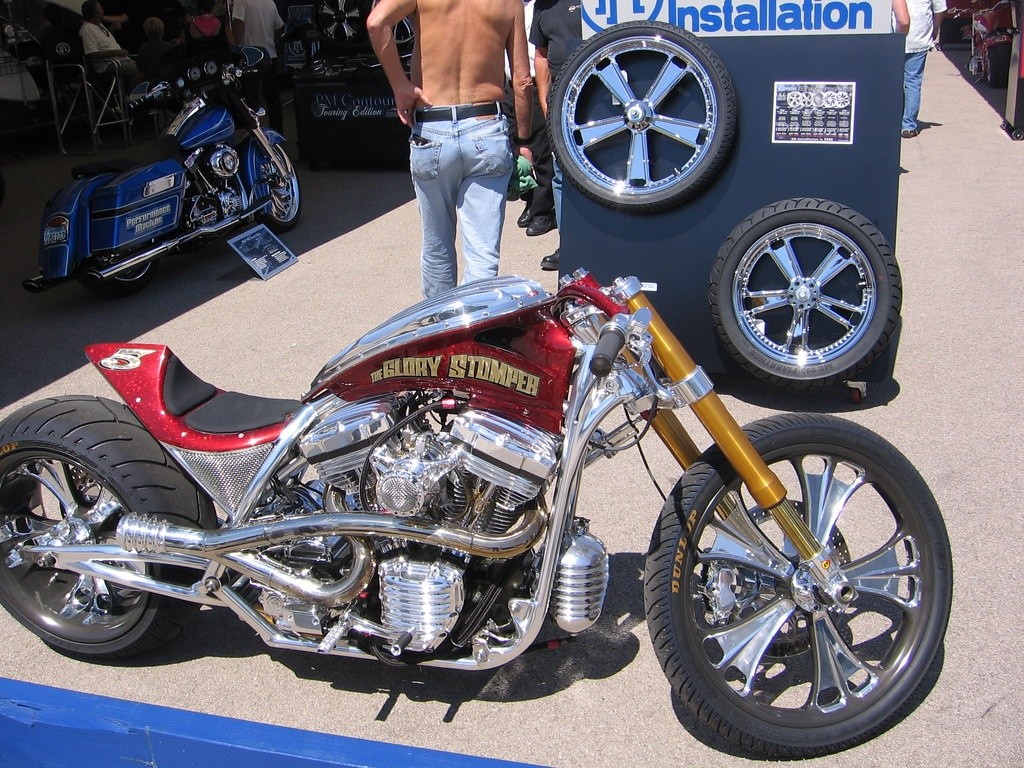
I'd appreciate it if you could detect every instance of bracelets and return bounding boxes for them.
[514,134,533,145]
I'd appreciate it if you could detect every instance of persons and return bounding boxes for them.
[368,0,535,304]
[505,0,946,271]
[35,0,286,149]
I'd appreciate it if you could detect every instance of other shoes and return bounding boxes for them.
[901,130,916,137]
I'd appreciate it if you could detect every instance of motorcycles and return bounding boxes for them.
[21,35,301,299]
[0,266,956,762]
[947,0,1013,90]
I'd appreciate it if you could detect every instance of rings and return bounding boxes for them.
[403,110,408,114]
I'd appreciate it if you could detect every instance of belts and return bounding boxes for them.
[415,103,503,123]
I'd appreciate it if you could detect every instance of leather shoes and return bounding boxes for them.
[518,206,535,227]
[541,248,560,268]
[526,215,556,235]
